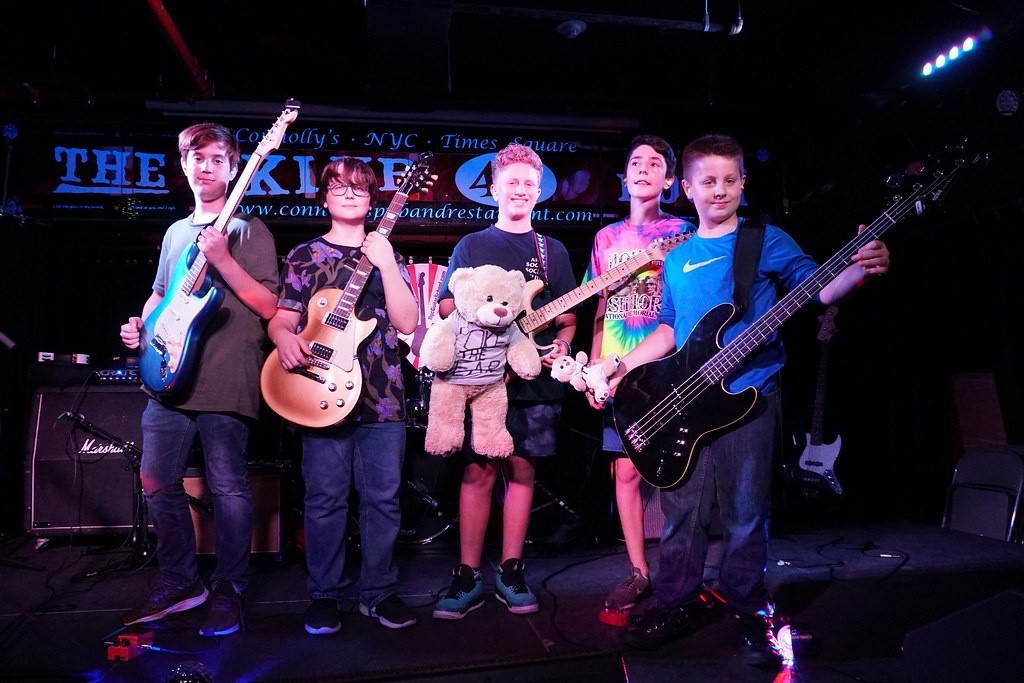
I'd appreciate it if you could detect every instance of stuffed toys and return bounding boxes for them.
[550,351,621,405]
[421,264,542,458]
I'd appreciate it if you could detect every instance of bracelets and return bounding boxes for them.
[560,339,571,356]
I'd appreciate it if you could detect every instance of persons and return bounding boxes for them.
[416,144,580,618]
[119,121,281,637]
[589,135,696,626]
[266,156,419,633]
[578,136,890,670]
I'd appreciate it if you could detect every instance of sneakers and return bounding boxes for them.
[493,558,539,613]
[198,580,245,636]
[737,627,783,666]
[359,594,417,628]
[432,563,486,620]
[624,607,693,650]
[121,577,210,626]
[305,599,342,634]
[604,567,652,609]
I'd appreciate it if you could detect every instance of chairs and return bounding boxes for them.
[940,446,1024,543]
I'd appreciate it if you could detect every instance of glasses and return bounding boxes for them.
[326,181,370,197]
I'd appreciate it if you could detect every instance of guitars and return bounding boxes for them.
[499,228,697,386]
[613,134,987,493]
[788,306,844,501]
[261,150,437,429]
[135,98,301,395]
